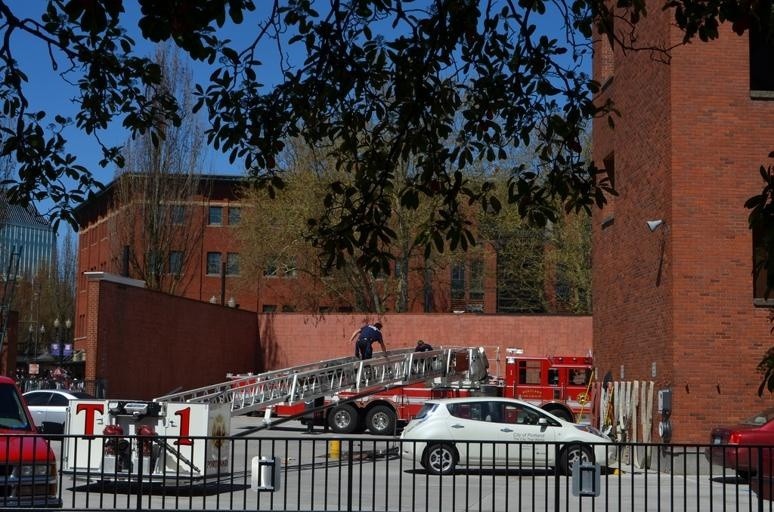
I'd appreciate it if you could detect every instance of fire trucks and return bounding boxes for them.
[62,346,593,486]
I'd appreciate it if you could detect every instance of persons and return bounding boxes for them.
[15,366,85,394]
[350,323,387,360]
[414,340,436,371]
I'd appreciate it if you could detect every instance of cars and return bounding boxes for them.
[398,397,617,476]
[705,408,774,499]
[0,376,79,506]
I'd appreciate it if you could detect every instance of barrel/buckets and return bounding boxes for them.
[329,440,340,454]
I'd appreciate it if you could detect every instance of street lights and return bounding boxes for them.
[29,324,45,361]
[54,318,71,354]
